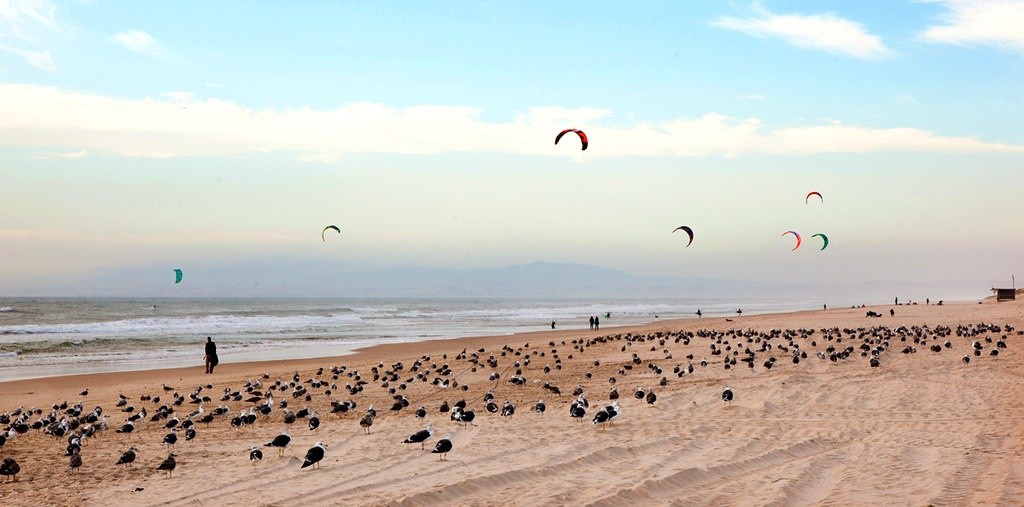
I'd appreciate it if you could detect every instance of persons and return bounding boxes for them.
[551,320,556,329]
[153,304,157,308]
[736,308,743,313]
[824,303,827,310]
[589,316,600,330]
[604,312,612,319]
[695,309,703,317]
[204,337,219,374]
[894,297,944,305]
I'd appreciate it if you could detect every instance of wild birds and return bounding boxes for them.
[501,401,515,422]
[431,433,452,461]
[722,384,733,411]
[249,446,263,461]
[263,431,292,458]
[439,401,450,416]
[301,442,328,470]
[536,400,545,419]
[1016,329,1024,335]
[487,399,498,418]
[635,387,644,402]
[0,322,1016,484]
[400,423,434,451]
[360,414,373,434]
[646,387,657,407]
[368,404,375,417]
[483,389,494,401]
[416,404,426,424]
[309,410,320,435]
